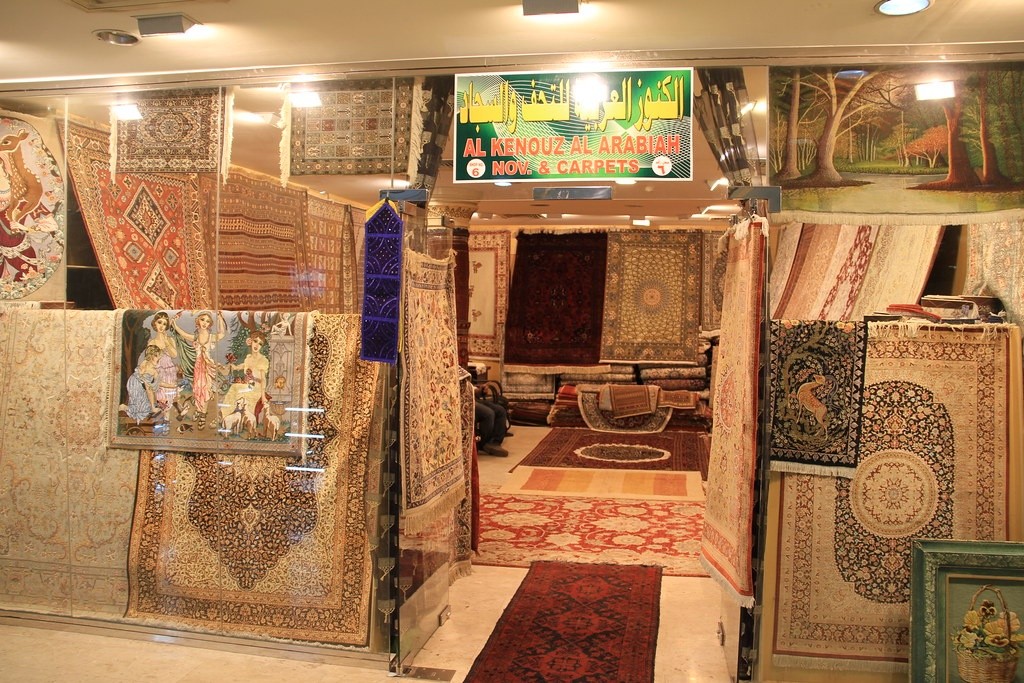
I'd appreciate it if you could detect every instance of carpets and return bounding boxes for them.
[0,74,1024,683]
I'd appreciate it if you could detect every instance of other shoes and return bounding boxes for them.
[478,442,508,456]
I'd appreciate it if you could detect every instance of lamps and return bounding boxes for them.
[129,13,203,37]
[522,0,581,16]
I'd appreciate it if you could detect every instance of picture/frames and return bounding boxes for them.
[911,539,1024,683]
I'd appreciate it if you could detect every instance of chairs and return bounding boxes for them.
[473,380,511,430]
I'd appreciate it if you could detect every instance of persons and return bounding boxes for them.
[472,381,513,458]
